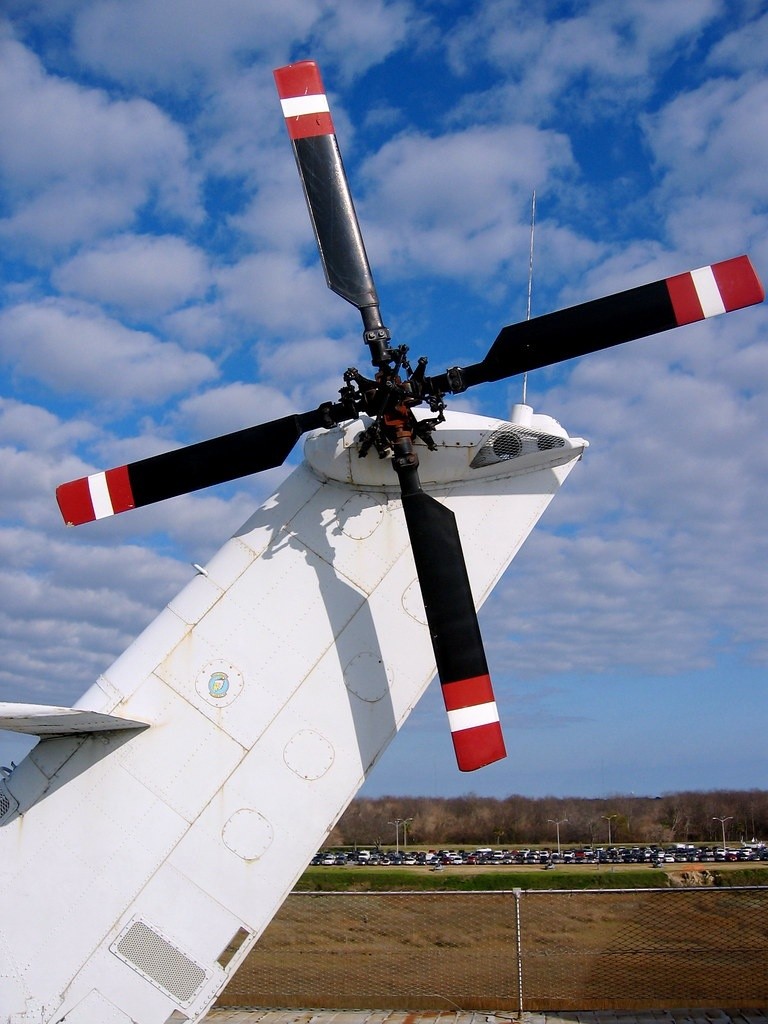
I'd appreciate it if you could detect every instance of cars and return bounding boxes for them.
[308,843,768,870]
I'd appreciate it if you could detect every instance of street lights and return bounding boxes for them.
[712,816,734,849]
[387,819,408,854]
[548,819,569,853]
[601,815,618,845]
[397,818,414,847]
